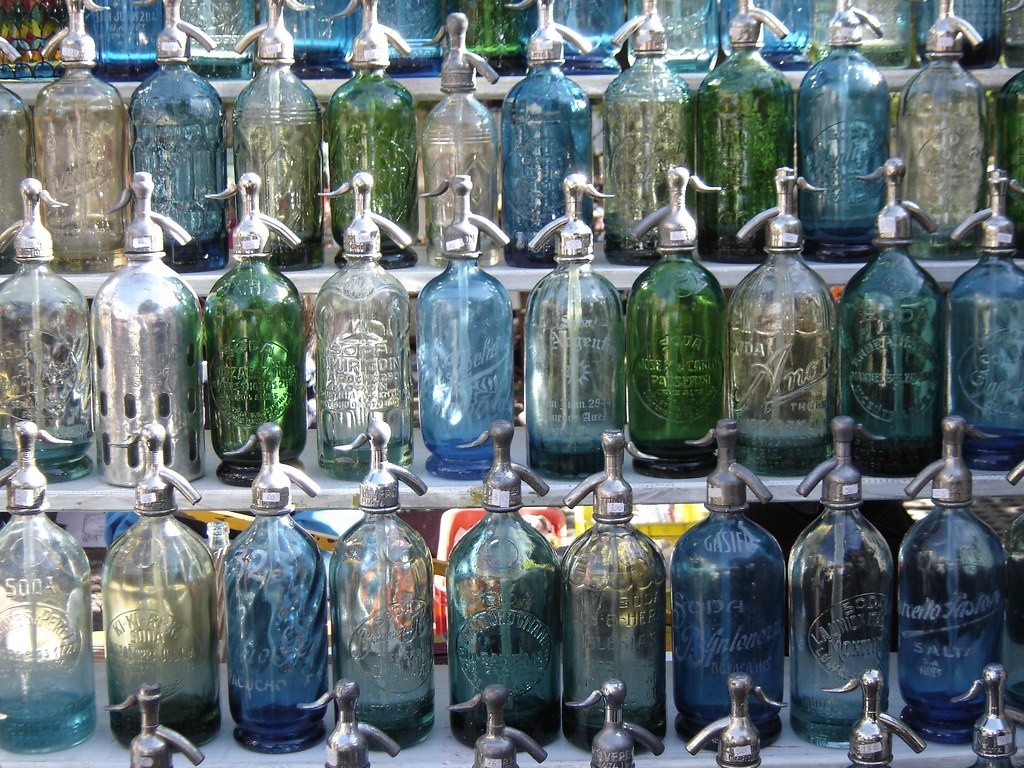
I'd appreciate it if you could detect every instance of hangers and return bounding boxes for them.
[0,67,1024,768]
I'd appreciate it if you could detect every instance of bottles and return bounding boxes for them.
[0,0,1024,768]
